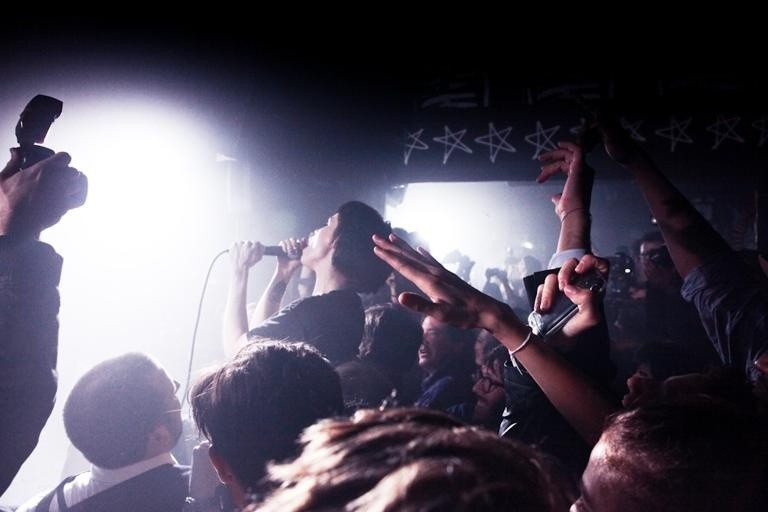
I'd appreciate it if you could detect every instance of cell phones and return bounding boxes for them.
[527,267,605,339]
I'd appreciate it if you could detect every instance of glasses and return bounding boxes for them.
[161,377,184,415]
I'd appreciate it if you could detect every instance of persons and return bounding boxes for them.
[1,146,88,492]
[189,137,768,512]
[33,353,192,512]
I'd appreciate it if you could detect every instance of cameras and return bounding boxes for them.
[11,93,64,176]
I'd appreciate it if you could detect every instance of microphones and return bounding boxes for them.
[242,238,301,261]
